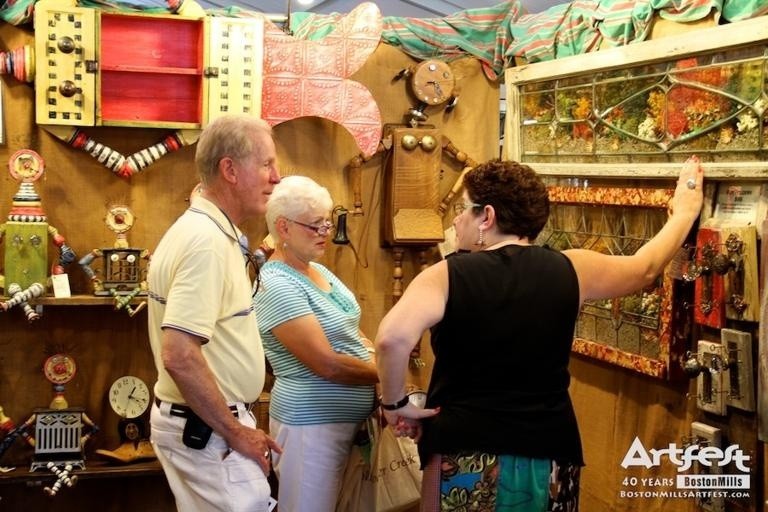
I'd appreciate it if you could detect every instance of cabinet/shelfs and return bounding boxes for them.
[30,7,263,133]
[0,292,166,483]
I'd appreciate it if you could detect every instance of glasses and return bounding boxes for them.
[286,219,336,236]
[453,202,480,215]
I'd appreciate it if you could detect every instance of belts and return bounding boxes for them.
[153,398,250,421]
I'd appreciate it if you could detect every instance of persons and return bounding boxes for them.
[147,111,288,512]
[373,153,704,512]
[251,173,376,512]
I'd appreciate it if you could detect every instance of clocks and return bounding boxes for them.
[108,374,151,420]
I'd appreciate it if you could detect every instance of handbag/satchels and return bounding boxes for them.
[338,417,426,512]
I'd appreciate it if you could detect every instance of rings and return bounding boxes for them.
[686,179,696,192]
[262,450,272,460]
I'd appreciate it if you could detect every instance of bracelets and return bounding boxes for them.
[379,395,411,411]
